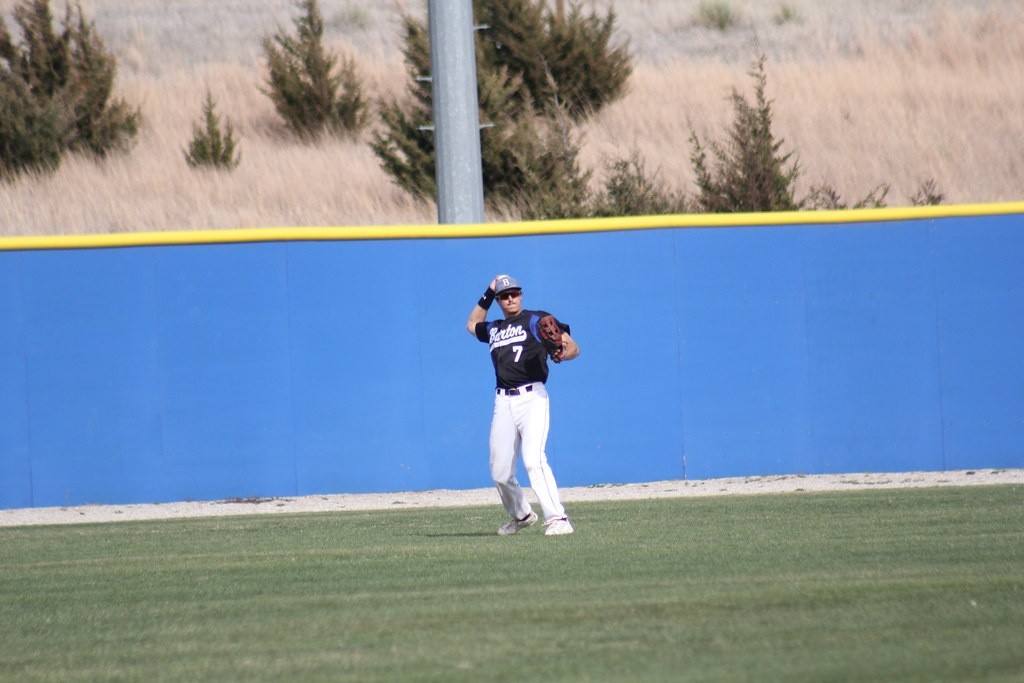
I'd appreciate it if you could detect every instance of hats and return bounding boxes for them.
[495,277,522,295]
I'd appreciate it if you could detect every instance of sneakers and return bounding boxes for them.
[498,511,538,536]
[541,518,574,535]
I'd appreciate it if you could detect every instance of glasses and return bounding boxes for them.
[496,292,522,300]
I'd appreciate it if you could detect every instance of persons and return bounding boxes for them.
[466,274,578,536]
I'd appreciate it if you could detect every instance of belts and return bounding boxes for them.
[497,385,532,395]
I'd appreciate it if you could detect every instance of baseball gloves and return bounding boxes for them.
[536,315,566,363]
